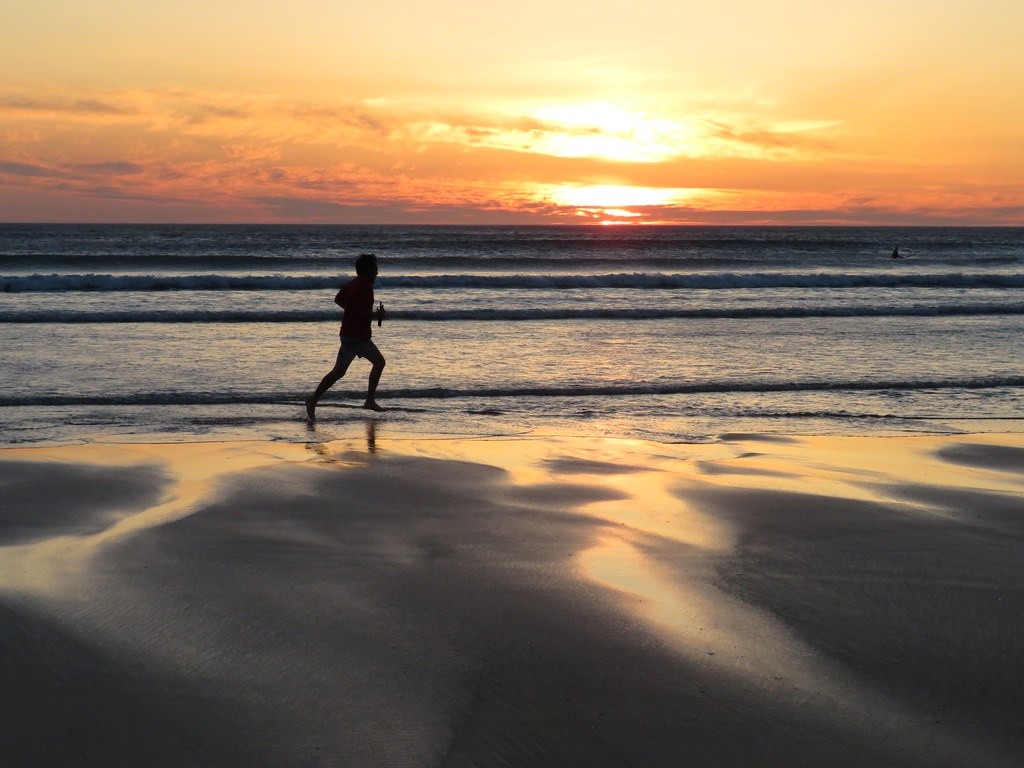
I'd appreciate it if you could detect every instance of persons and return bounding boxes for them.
[304,254,385,422]
